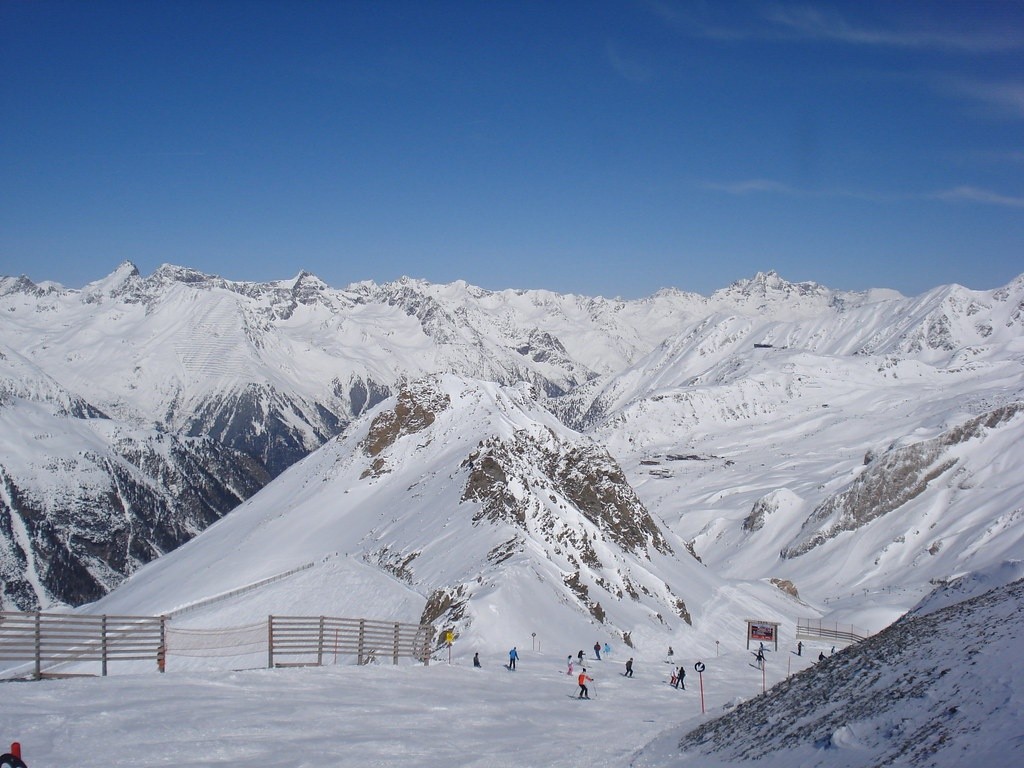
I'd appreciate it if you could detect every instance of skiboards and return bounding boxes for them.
[661,680,677,688]
[619,672,636,678]
[566,694,600,701]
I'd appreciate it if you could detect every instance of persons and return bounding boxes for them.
[578,668,594,700]
[831,646,835,653]
[624,658,633,678]
[798,641,804,656]
[594,642,601,660]
[567,655,574,676]
[671,666,686,690]
[665,647,674,664]
[819,652,826,661]
[508,647,519,670]
[756,641,766,669]
[603,642,610,656]
[578,650,586,665]
[473,652,481,668]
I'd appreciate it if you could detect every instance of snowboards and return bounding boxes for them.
[503,664,517,673]
